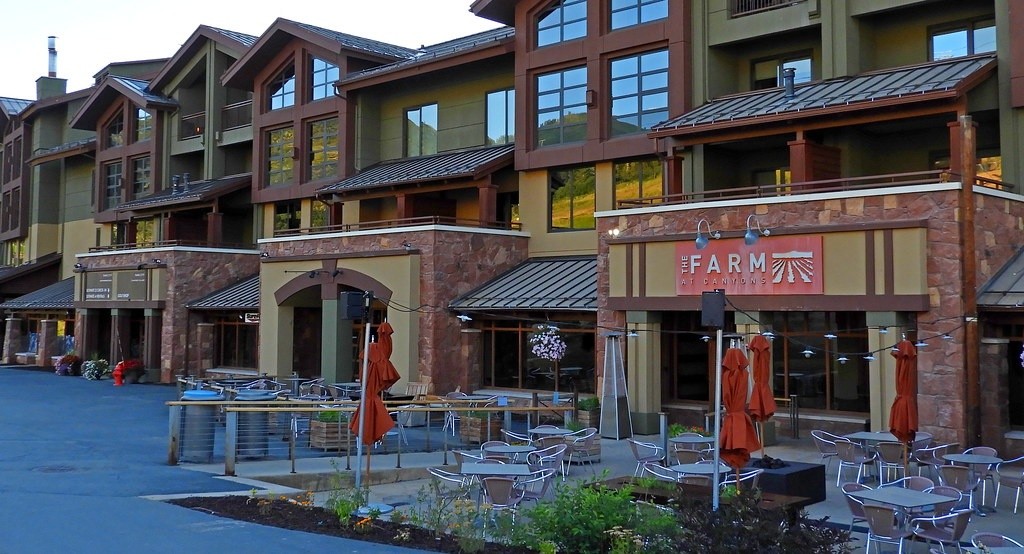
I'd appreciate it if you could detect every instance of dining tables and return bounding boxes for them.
[845,429,934,444]
[937,451,1008,466]
[461,463,534,476]
[529,428,575,435]
[960,546,1024,554]
[669,463,736,476]
[670,436,718,444]
[845,485,958,510]
[456,394,491,400]
[484,445,539,454]
[334,381,362,387]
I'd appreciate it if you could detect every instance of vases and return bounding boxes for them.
[91,371,104,381]
[125,369,141,384]
[65,367,78,376]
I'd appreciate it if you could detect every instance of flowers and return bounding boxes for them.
[54,354,82,377]
[81,354,108,381]
[112,360,145,387]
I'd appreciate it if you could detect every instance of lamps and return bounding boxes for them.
[745,215,770,246]
[696,219,720,250]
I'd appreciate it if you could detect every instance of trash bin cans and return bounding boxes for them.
[179,390,226,463]
[234,390,277,458]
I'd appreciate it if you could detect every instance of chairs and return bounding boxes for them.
[175,372,997,554]
[971,532,1024,548]
[994,456,1024,515]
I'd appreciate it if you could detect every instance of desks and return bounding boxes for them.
[582,475,809,517]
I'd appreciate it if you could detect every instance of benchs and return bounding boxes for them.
[740,458,826,508]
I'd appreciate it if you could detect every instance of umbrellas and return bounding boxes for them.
[720,339,762,491]
[749,330,777,457]
[349,335,395,483]
[889,332,918,477]
[377,318,401,400]
